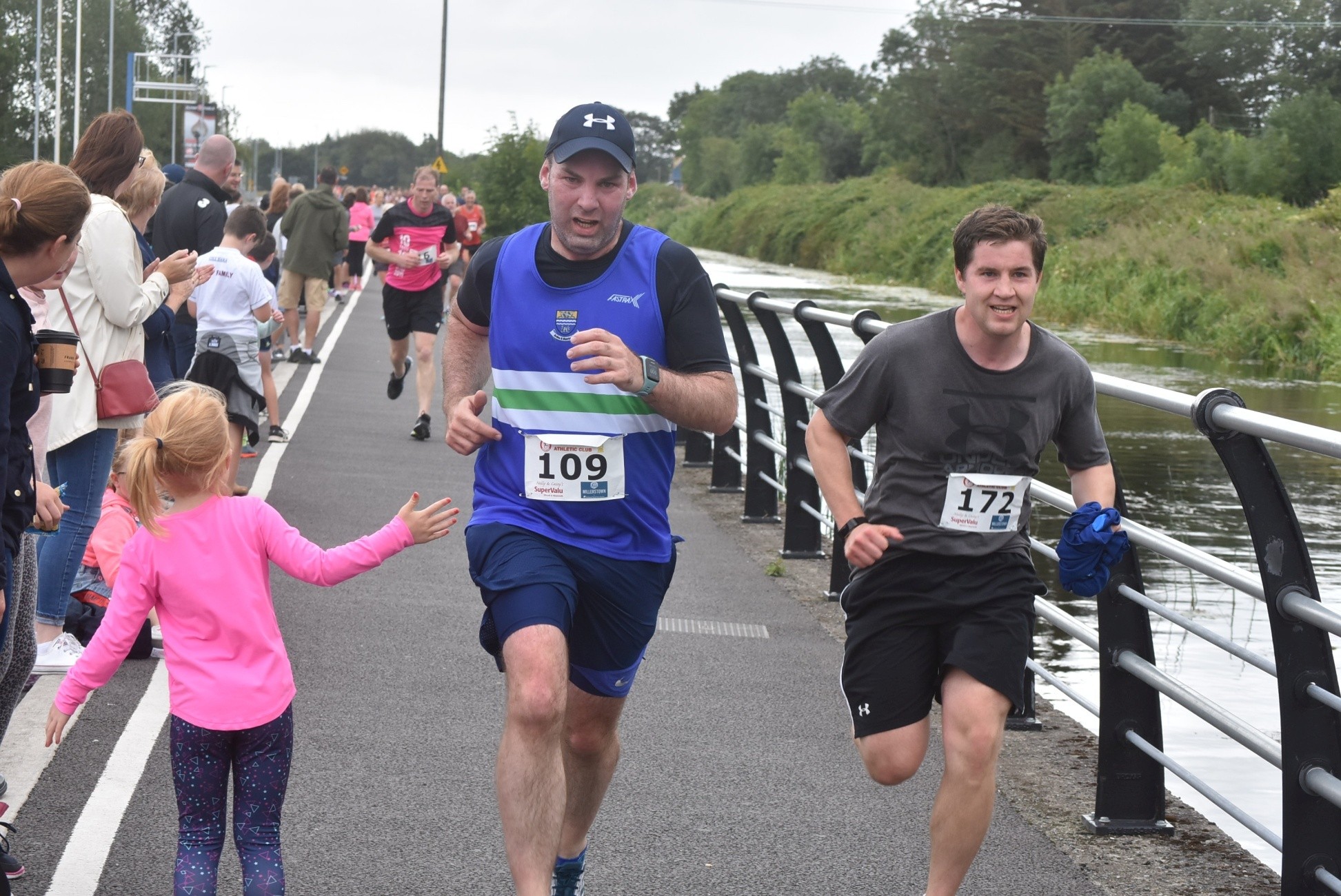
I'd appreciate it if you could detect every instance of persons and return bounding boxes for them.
[806,206,1121,896]
[32,110,289,673]
[443,102,737,896]
[0,155,91,896]
[44,385,461,896]
[260,164,488,365]
[365,166,460,441]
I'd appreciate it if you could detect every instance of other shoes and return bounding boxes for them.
[329,288,335,297]
[233,485,248,496]
[151,625,163,649]
[0,775,7,798]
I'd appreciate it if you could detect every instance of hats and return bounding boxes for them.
[544,101,637,174]
[162,164,186,184]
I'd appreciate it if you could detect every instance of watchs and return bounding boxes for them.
[632,355,660,397]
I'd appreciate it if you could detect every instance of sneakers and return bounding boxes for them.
[240,444,258,458]
[335,291,345,305]
[551,840,589,896]
[288,348,321,364]
[411,410,431,442]
[341,281,361,291]
[387,355,413,400]
[268,425,289,442]
[0,851,25,879]
[271,348,285,363]
[30,632,86,675]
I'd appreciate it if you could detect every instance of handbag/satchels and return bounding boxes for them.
[97,359,159,419]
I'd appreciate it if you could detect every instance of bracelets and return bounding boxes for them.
[836,517,868,541]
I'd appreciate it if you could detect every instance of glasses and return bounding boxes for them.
[136,156,146,168]
[230,172,245,177]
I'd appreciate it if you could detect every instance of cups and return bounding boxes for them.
[33,329,80,394]
